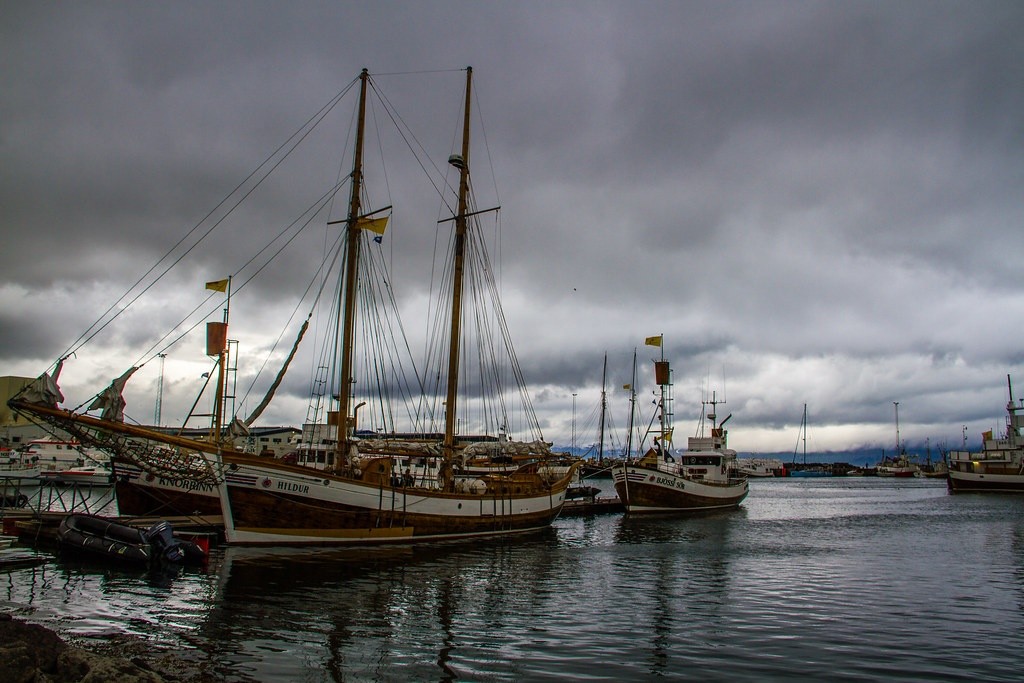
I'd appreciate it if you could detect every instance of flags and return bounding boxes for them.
[201,373,208,378]
[205,279,228,292]
[623,384,630,390]
[656,431,671,441]
[355,217,388,235]
[645,336,662,347]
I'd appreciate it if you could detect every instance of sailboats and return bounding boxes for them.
[914,438,948,478]
[944,373,1024,493]
[6,66,582,549]
[579,351,623,478]
[877,400,921,476]
[790,403,833,477]
[611,331,754,513]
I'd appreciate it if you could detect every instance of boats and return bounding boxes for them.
[741,457,774,477]
[45,509,203,576]
[40,465,113,485]
[0,462,40,484]
[847,470,864,476]
[10,439,111,468]
